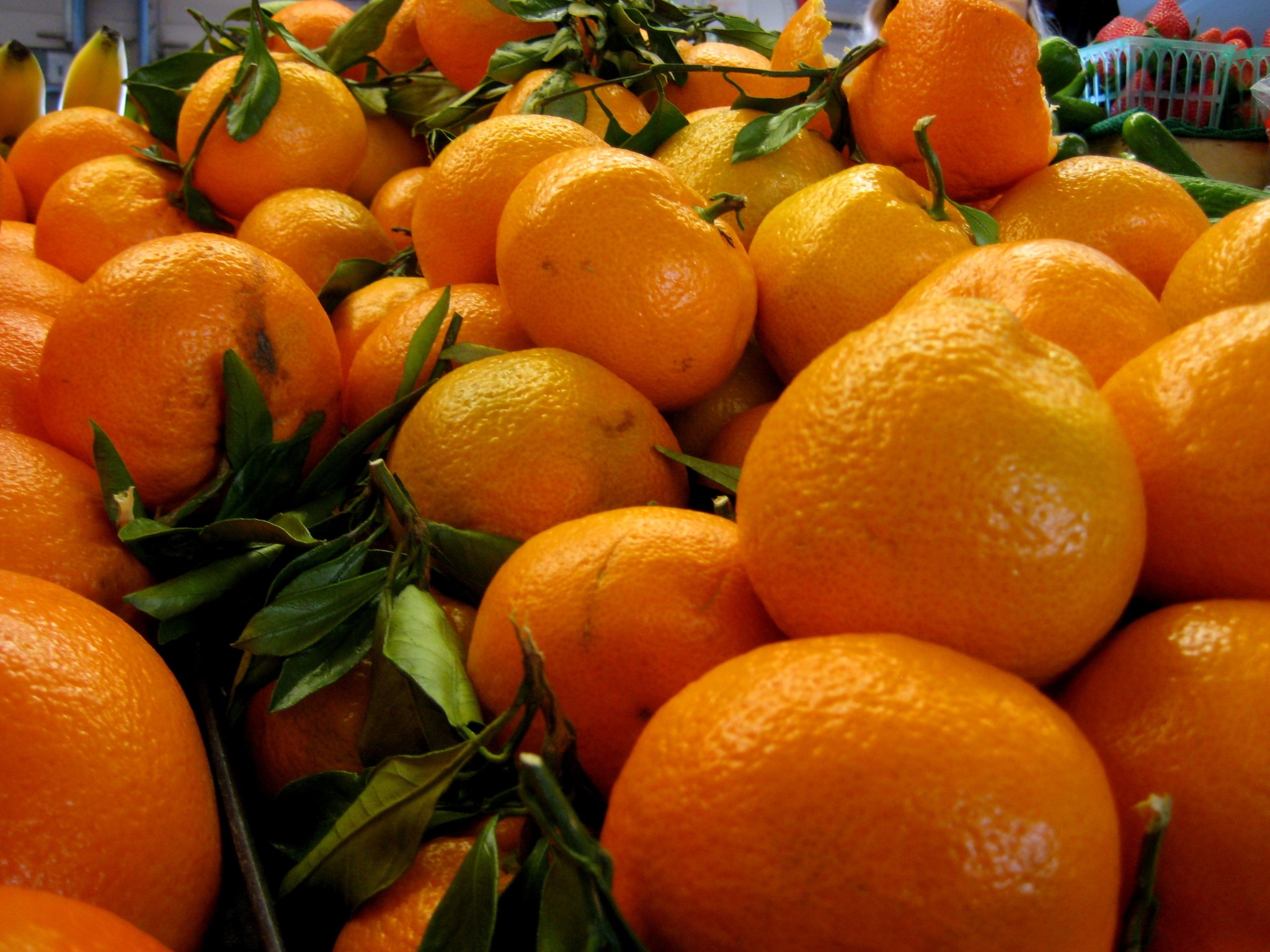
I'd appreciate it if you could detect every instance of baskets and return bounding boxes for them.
[1078,36,1237,130]
[1228,48,1269,136]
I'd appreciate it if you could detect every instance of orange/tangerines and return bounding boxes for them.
[1,3,1267,952]
